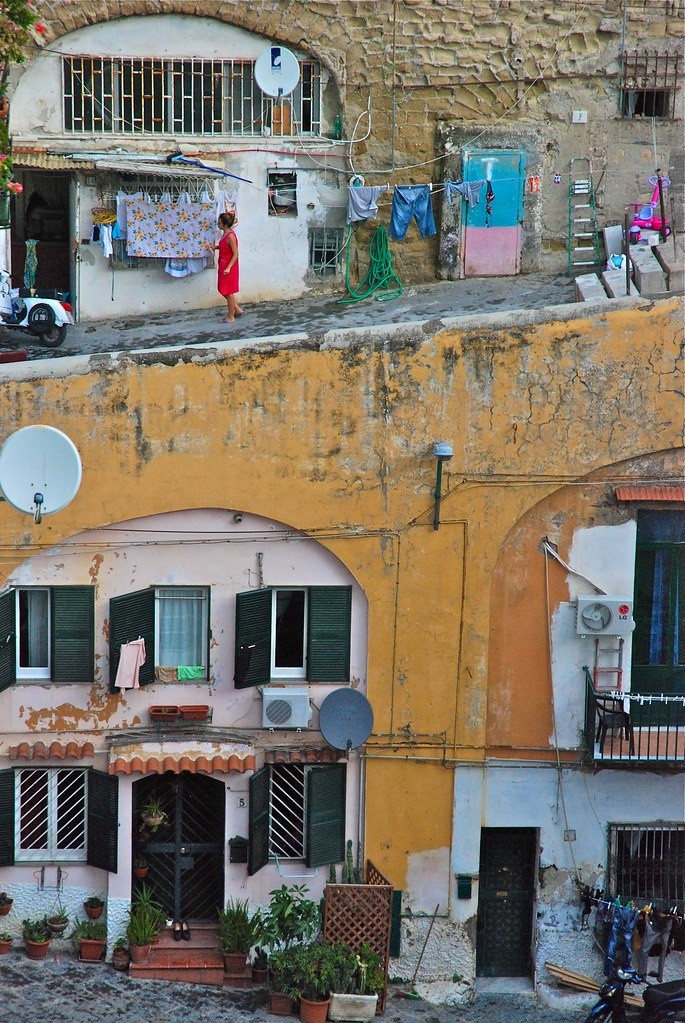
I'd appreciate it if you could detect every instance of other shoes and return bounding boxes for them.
[174,921,190,941]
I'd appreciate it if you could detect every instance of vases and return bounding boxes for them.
[0,190,10,226]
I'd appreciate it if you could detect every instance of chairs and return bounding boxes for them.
[595,695,635,756]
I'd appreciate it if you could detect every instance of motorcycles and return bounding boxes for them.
[0,270,76,345]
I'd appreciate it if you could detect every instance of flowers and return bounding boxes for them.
[0,0,45,62]
[0,118,23,193]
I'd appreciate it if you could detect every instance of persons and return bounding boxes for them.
[206,213,244,323]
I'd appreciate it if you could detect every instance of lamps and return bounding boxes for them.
[433,443,454,530]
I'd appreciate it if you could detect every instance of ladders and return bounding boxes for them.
[595,637,625,739]
[568,158,602,275]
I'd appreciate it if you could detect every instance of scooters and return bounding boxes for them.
[585,960,685,1022]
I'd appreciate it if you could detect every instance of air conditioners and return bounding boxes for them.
[262,688,312,727]
[574,594,633,636]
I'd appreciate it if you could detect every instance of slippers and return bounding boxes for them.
[219,317,236,323]
[234,312,244,316]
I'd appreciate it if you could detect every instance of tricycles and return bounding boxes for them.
[623,173,671,246]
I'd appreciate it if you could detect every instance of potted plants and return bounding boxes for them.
[138,794,168,832]
[113,881,167,971]
[0,81,10,117]
[215,884,385,1023]
[83,897,104,919]
[63,916,107,962]
[132,855,150,877]
[47,905,71,932]
[0,892,13,915]
[180,705,209,720]
[150,706,179,722]
[22,914,53,959]
[0,932,13,954]
